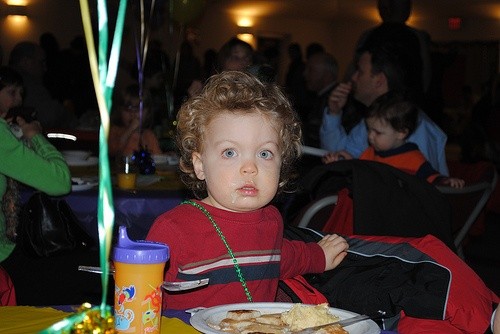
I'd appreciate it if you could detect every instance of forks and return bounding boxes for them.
[78,266,209,291]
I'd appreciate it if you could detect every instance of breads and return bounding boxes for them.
[216,308,290,334]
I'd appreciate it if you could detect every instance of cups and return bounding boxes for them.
[115,161,137,191]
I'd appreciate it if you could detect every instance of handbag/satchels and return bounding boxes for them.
[19,195,94,258]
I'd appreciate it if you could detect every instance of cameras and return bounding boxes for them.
[4,105,38,125]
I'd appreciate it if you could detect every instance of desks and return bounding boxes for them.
[22,164,209,243]
[0,305,401,334]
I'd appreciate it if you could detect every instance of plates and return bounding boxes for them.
[189,302,381,334]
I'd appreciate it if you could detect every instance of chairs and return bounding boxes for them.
[0,146,500,334]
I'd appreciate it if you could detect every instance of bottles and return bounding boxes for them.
[112,226,171,334]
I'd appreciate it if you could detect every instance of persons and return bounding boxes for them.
[0,31,99,264]
[109,0,500,191]
[146,70,349,310]
[0,266,17,306]
